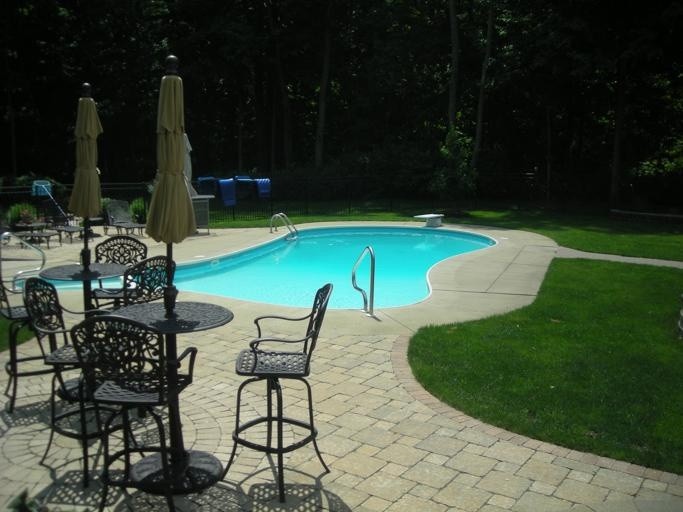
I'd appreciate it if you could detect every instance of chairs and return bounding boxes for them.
[86,254,176,357]
[89,235,148,313]
[0,200,144,248]
[0,248,72,413]
[21,276,144,486]
[219,282,334,502]
[69,314,198,512]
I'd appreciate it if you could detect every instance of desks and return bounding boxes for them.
[92,300,234,492]
[39,261,131,338]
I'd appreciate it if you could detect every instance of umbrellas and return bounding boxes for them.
[143,54,198,285]
[65,82,105,251]
